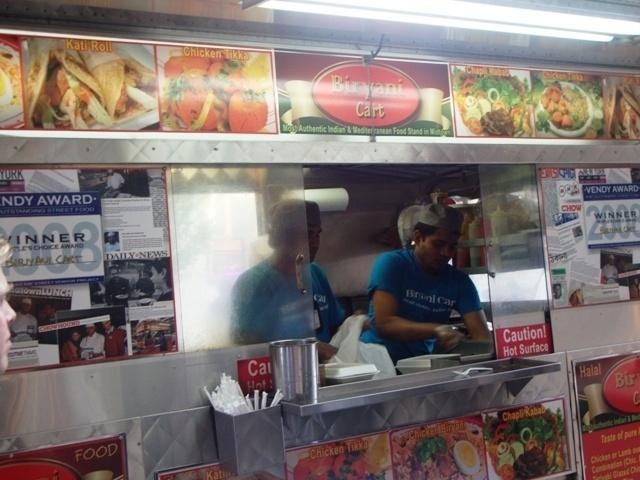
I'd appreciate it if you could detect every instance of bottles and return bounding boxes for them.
[457,205,510,268]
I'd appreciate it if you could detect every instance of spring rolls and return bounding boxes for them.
[607,77,640,140]
[27,39,156,130]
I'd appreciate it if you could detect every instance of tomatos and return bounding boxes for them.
[164,49,269,133]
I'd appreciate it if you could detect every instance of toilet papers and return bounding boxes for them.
[286,187,349,214]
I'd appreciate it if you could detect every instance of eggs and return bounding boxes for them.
[0,68,14,108]
[454,440,480,474]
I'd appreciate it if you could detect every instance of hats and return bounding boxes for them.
[418,204,464,234]
[272,199,321,230]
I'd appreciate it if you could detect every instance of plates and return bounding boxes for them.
[539,81,594,136]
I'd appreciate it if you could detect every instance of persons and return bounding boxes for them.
[229,199,370,364]
[105,169,125,192]
[61,320,127,362]
[601,254,618,284]
[360,204,493,366]
[10,298,37,341]
[104,262,173,305]
